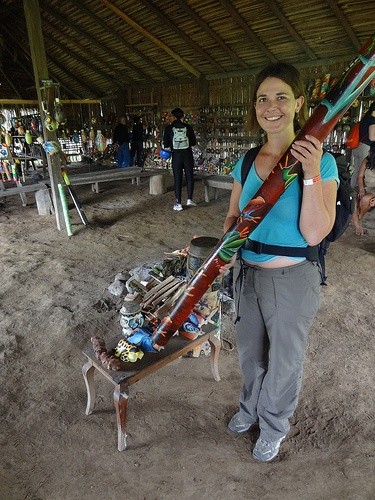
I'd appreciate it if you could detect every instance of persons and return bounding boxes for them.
[13,126,31,177]
[347,101,375,234]
[164,108,197,211]
[112,114,145,170]
[219,60,340,462]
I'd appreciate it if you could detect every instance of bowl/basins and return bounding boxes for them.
[180,327,200,340]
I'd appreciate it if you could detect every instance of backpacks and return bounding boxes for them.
[345,117,373,149]
[241,145,355,262]
[170,123,189,149]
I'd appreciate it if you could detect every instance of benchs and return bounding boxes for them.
[38,167,141,194]
[202,174,234,202]
[0,184,47,198]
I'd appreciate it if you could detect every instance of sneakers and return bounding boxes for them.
[228,411,257,433]
[252,424,290,462]
[186,199,197,207]
[172,204,183,211]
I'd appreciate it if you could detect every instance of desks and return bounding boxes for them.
[80,323,221,452]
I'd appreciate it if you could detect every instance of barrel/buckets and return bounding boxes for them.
[186,236,222,292]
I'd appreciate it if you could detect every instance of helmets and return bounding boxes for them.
[160,147,172,159]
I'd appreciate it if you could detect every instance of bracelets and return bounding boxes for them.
[303,175,322,186]
